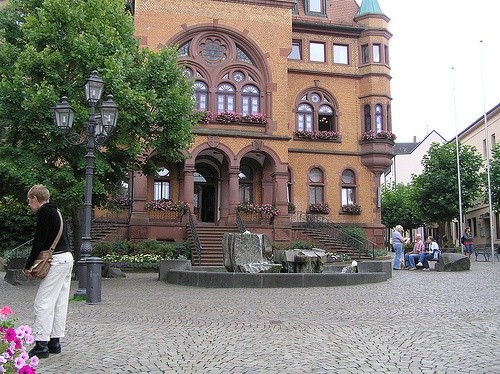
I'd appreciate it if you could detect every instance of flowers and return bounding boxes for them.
[244,112,268,124]
[214,110,242,125]
[197,109,213,124]
[341,203,362,215]
[146,198,190,216]
[362,130,397,144]
[309,201,331,215]
[236,201,281,219]
[295,130,340,141]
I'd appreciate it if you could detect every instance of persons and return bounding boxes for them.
[464,226,474,258]
[392,226,408,270]
[404,234,425,269]
[416,237,440,271]
[194,190,199,211]
[24,184,73,359]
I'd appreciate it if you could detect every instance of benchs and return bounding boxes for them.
[473,243,500,261]
[423,249,455,262]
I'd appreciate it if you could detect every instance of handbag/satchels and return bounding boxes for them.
[22,250,53,278]
[432,249,441,260]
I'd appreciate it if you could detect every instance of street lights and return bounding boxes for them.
[49,70,120,301]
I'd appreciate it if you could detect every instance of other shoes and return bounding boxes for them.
[27,341,49,359]
[47,338,61,354]
[409,267,416,271]
[423,267,430,271]
[404,266,410,270]
[416,262,423,266]
